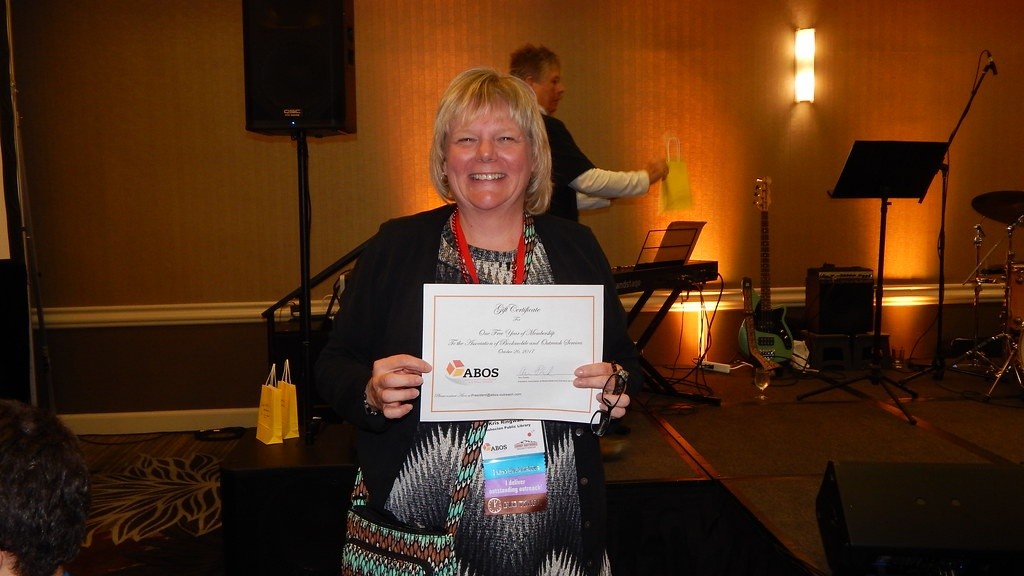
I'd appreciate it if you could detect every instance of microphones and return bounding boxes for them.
[986,51,997,75]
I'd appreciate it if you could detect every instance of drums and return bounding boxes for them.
[1004,260,1024,375]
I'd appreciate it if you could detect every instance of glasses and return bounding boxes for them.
[591,361,627,438]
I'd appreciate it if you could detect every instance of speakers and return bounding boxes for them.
[240,0,358,137]
[815,458,1024,576]
[217,461,354,576]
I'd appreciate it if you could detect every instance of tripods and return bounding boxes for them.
[798,66,1024,426]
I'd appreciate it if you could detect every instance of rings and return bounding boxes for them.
[618,370,628,386]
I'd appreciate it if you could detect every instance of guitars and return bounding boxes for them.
[735,174,795,365]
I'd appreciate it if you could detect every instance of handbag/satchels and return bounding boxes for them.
[277,359,299,439]
[255,363,283,445]
[341,506,456,576]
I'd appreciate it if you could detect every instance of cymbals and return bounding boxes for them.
[970,190,1024,227]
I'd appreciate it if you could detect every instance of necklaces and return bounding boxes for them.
[452,206,519,284]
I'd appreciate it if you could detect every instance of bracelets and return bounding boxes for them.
[363,391,384,417]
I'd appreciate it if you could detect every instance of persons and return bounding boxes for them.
[510,43,668,438]
[312,67,640,576]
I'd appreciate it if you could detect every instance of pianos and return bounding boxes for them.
[610,259,719,295]
